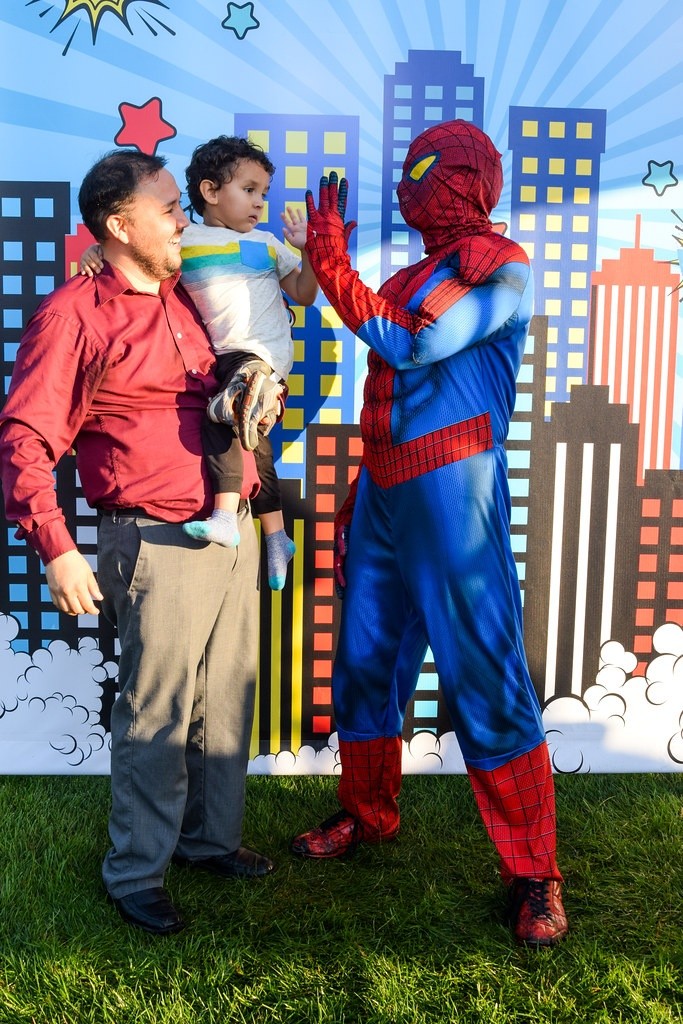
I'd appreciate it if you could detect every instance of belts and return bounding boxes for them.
[103,507,149,519]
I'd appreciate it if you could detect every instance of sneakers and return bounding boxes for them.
[237,371,284,451]
[206,359,272,422]
[292,809,398,857]
[512,876,567,946]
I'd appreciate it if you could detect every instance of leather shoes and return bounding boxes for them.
[117,888,181,935]
[173,847,273,879]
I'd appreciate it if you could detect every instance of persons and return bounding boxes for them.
[291,118,568,946]
[0,149,288,935]
[79,134,321,593]
